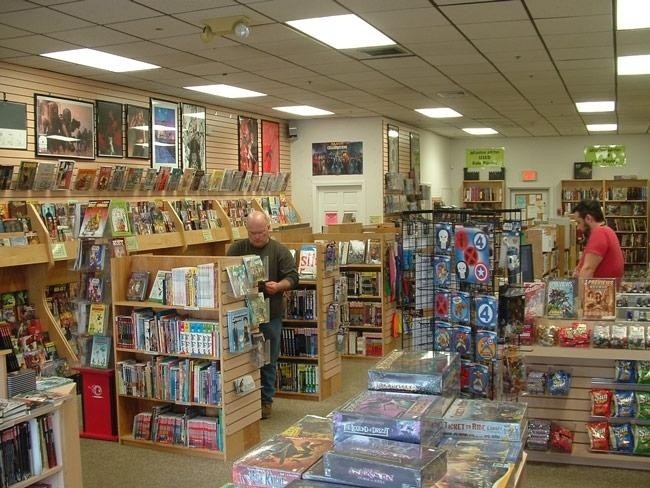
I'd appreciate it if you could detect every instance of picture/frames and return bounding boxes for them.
[33,91,208,173]
[237,114,281,177]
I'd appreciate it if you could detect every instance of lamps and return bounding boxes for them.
[232,22,251,44]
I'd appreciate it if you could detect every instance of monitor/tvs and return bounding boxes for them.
[521,246,534,282]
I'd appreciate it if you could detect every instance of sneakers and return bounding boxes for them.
[259,401,273,420]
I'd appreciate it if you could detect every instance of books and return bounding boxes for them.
[564,186,648,271]
[73,238,106,272]
[117,254,269,451]
[464,185,501,202]
[299,239,384,394]
[0,161,297,247]
[0,280,111,487]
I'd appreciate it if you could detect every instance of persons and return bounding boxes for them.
[226,210,299,418]
[573,198,624,280]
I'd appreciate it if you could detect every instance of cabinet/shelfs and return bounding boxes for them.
[0,178,650,488]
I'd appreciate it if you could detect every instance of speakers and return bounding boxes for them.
[288,121,298,136]
